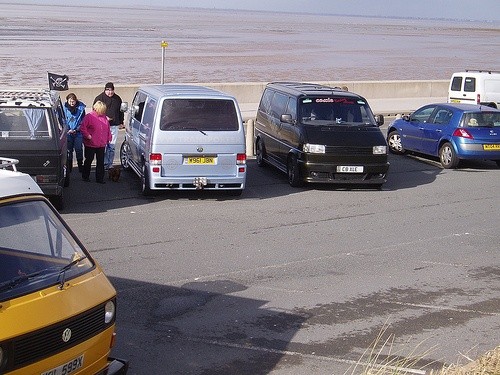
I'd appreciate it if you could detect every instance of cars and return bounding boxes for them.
[387,103,500,169]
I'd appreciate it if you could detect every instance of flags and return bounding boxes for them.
[47,73,68,91]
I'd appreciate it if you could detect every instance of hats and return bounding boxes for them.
[105,82,114,91]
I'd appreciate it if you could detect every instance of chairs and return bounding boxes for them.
[439,112,449,121]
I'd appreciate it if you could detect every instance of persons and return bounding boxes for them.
[79,101,112,183]
[93,83,125,171]
[62,93,86,173]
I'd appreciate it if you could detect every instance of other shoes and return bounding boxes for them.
[96,179,106,184]
[104,166,108,171]
[108,165,115,169]
[83,178,90,182]
[78,166,82,172]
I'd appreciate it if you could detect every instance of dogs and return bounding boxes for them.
[107,166,122,183]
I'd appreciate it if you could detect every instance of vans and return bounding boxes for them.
[0,88,74,202]
[0,155,130,375]
[447,69,500,121]
[255,81,391,190]
[120,84,247,197]
